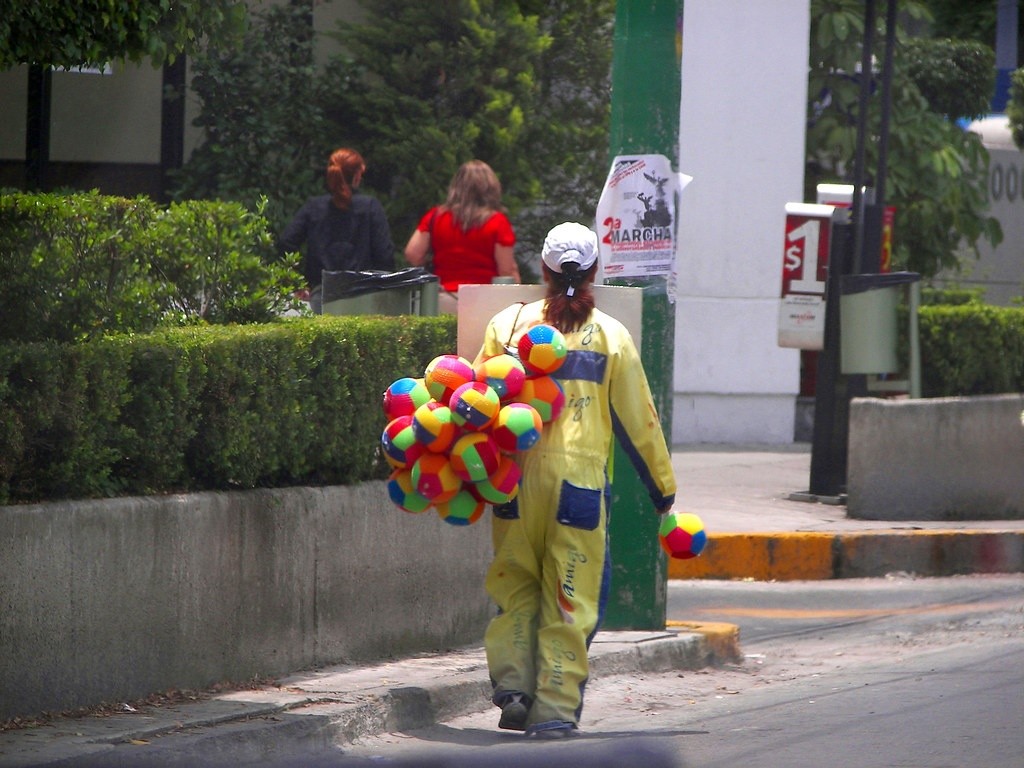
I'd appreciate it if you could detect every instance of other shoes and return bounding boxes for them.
[536,728,565,739]
[499,693,531,731]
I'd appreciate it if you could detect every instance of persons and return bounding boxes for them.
[470,221,677,739]
[271,148,397,315]
[402,157,520,318]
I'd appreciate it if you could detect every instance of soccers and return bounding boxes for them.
[658,511,707,560]
[379,322,569,526]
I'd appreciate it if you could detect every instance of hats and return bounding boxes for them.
[542,222,598,274]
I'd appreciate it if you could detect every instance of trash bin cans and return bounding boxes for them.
[320,267,440,319]
[836,270,922,374]
